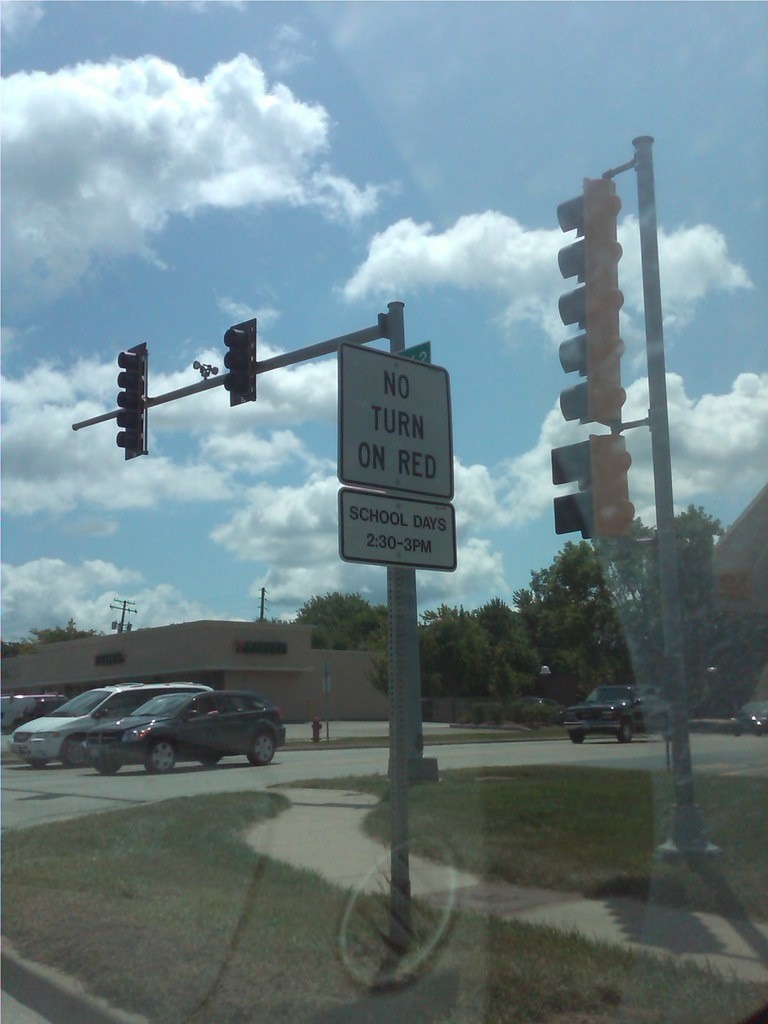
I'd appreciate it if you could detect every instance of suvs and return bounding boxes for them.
[562,684,674,743]
[79,691,286,775]
[7,678,215,769]
[1,693,71,752]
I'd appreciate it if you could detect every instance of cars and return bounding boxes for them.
[730,702,768,735]
[513,695,558,708]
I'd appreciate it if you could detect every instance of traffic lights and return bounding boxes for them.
[548,433,635,542]
[554,175,627,427]
[222,318,259,407]
[115,343,151,461]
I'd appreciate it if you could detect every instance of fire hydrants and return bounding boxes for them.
[310,716,324,744]
